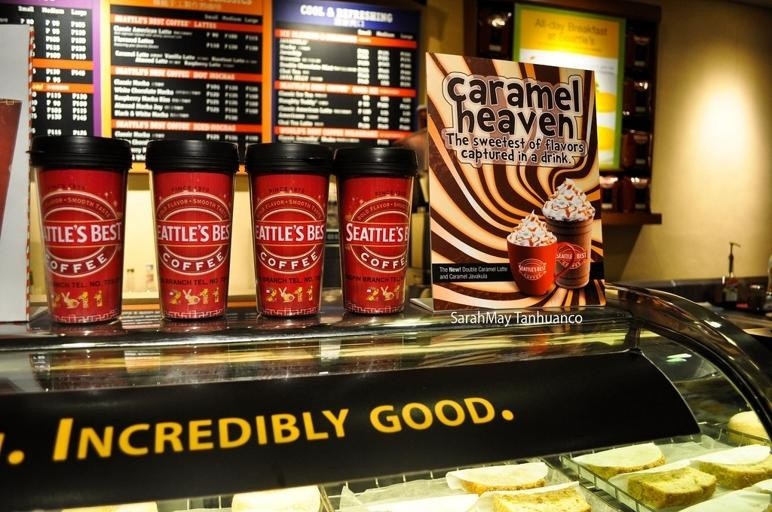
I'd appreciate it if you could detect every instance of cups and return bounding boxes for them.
[510,236,575,296]
[746,285,764,306]
[25,136,419,323]
[545,216,592,287]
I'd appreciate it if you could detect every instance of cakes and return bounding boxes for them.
[442,461,595,511]
[573,409,772,512]
[231,483,322,512]
